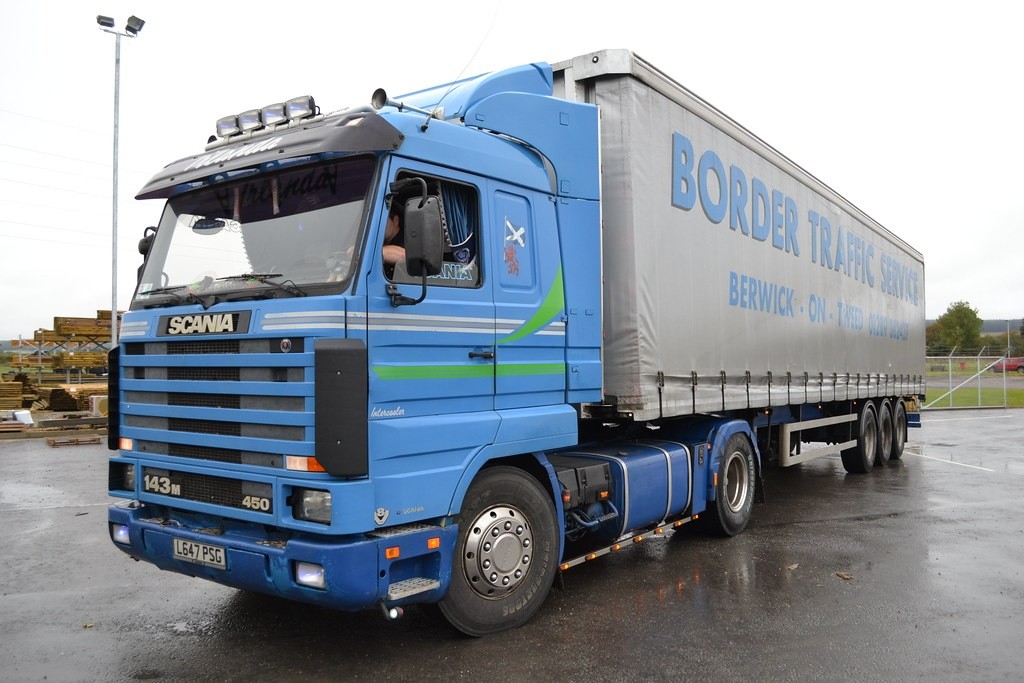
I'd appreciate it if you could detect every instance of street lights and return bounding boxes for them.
[94,13,146,351]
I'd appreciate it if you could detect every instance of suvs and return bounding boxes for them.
[987,357,1024,374]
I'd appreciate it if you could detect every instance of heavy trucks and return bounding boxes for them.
[105,46,929,640]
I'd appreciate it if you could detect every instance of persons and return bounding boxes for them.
[346,205,406,281]
